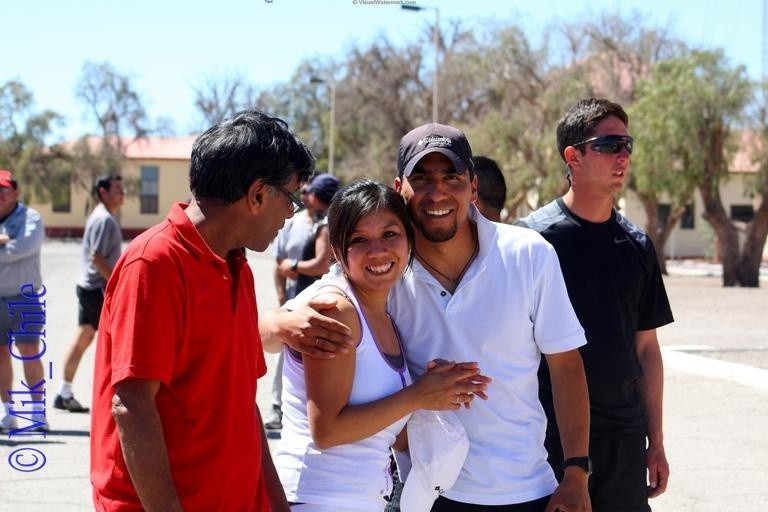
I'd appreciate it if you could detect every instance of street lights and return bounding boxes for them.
[402,5,440,125]
[309,77,335,177]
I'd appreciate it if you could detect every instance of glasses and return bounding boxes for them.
[270,182,306,214]
[571,135,636,155]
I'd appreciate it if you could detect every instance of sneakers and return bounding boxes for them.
[52,392,91,415]
[30,406,51,432]
[1,410,19,435]
[263,404,284,431]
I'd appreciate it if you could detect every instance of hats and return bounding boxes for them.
[397,122,476,179]
[302,173,340,193]
[0,169,17,191]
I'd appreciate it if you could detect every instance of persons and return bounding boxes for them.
[256,98,675,511]
[53,172,125,414]
[0,168,49,433]
[89,109,318,512]
